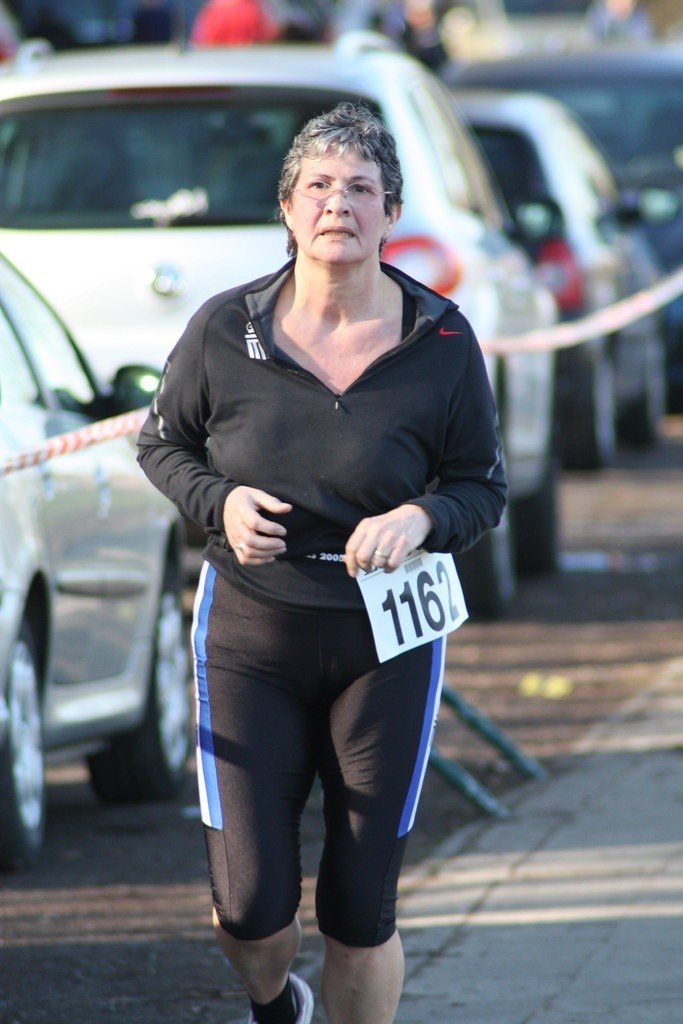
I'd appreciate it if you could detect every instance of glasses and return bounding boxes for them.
[292,181,393,209]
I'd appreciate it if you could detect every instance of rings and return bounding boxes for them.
[375,550,389,559]
[239,543,245,552]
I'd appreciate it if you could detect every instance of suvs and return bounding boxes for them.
[0,30,569,624]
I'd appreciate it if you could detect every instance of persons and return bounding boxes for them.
[136,102,508,1024]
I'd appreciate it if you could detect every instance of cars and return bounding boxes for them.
[435,36,682,416]
[0,235,201,872]
[441,85,682,477]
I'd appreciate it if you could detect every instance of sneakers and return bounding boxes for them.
[248,972,314,1024]
